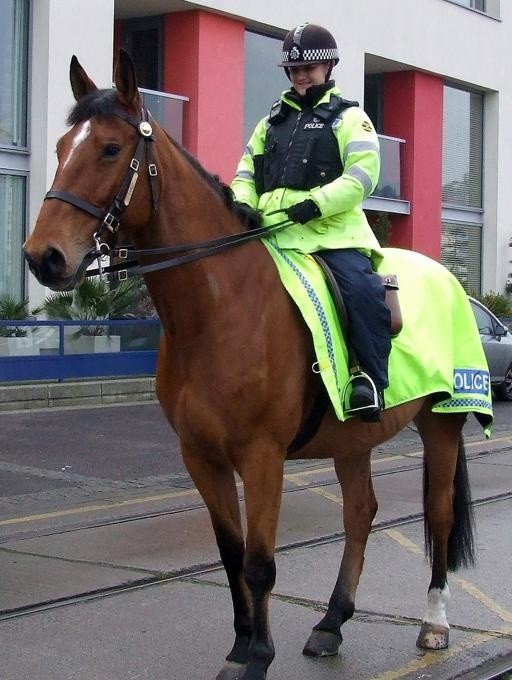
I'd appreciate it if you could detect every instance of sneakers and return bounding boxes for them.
[350,386,385,423]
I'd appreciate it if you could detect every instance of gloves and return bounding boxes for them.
[233,202,263,228]
[284,199,322,224]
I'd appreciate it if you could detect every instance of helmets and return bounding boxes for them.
[278,22,339,68]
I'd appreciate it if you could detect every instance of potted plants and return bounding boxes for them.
[1,276,147,358]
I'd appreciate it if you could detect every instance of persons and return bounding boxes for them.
[229,21,391,423]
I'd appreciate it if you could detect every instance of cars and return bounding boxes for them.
[465,294,512,403]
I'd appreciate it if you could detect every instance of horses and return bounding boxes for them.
[18,54,479,680]
